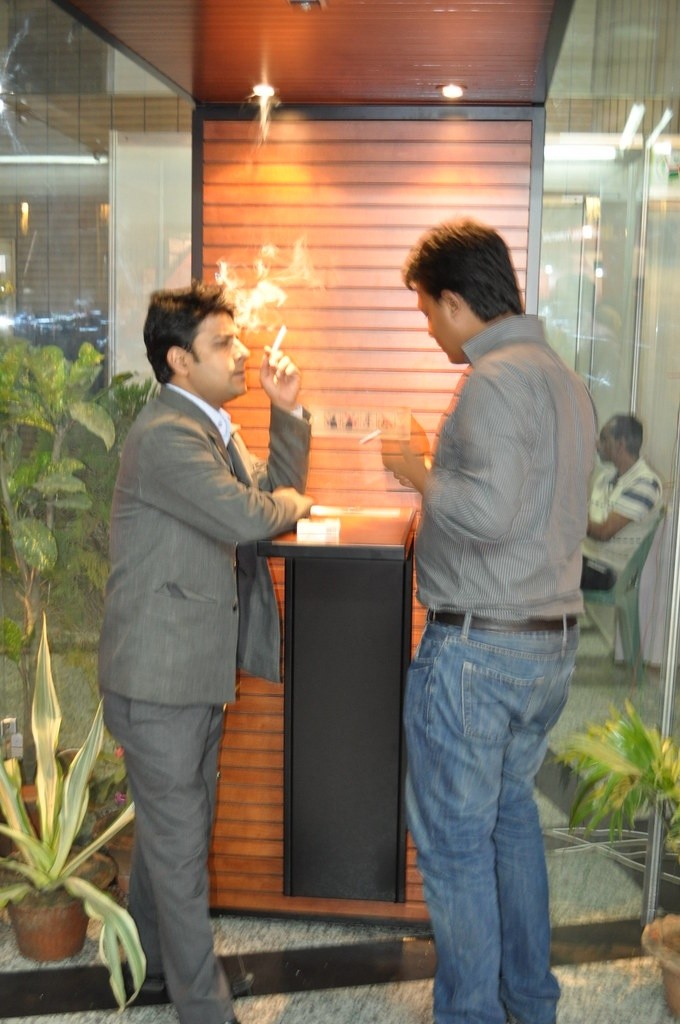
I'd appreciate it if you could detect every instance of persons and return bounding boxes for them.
[380,220,594,1024]
[100,287,315,1024]
[580,415,663,589]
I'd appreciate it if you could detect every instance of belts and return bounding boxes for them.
[427,609,577,631]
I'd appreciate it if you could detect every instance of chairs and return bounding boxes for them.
[584,507,666,687]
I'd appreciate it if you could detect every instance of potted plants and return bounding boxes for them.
[0,332,135,857]
[0,612,147,1015]
[548,695,680,1018]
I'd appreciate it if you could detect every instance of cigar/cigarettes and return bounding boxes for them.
[359,429,382,444]
[271,326,286,354]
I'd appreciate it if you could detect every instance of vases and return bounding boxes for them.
[88,811,136,895]
